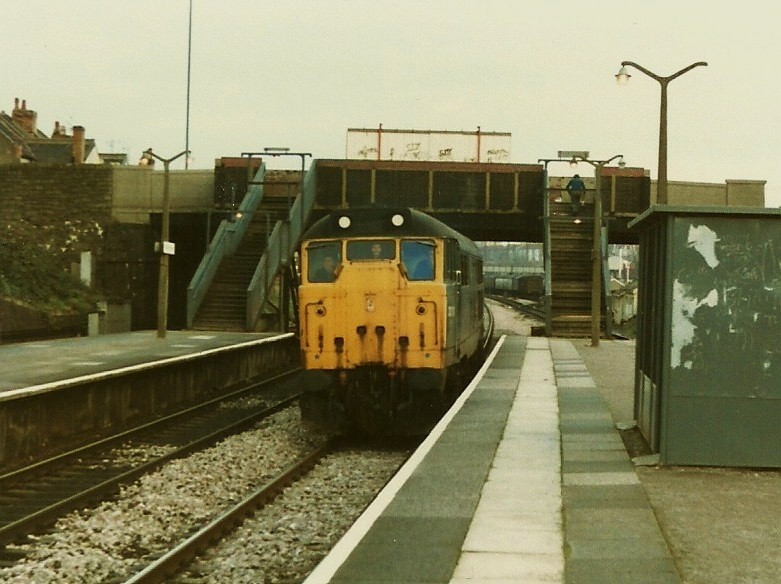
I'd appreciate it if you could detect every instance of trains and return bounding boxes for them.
[297,203,484,437]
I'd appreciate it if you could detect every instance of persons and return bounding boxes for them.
[566,174,586,216]
[413,250,435,280]
[371,241,383,258]
[314,253,338,283]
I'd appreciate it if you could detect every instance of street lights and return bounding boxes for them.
[141,148,197,338]
[570,155,627,347]
[614,61,718,203]
